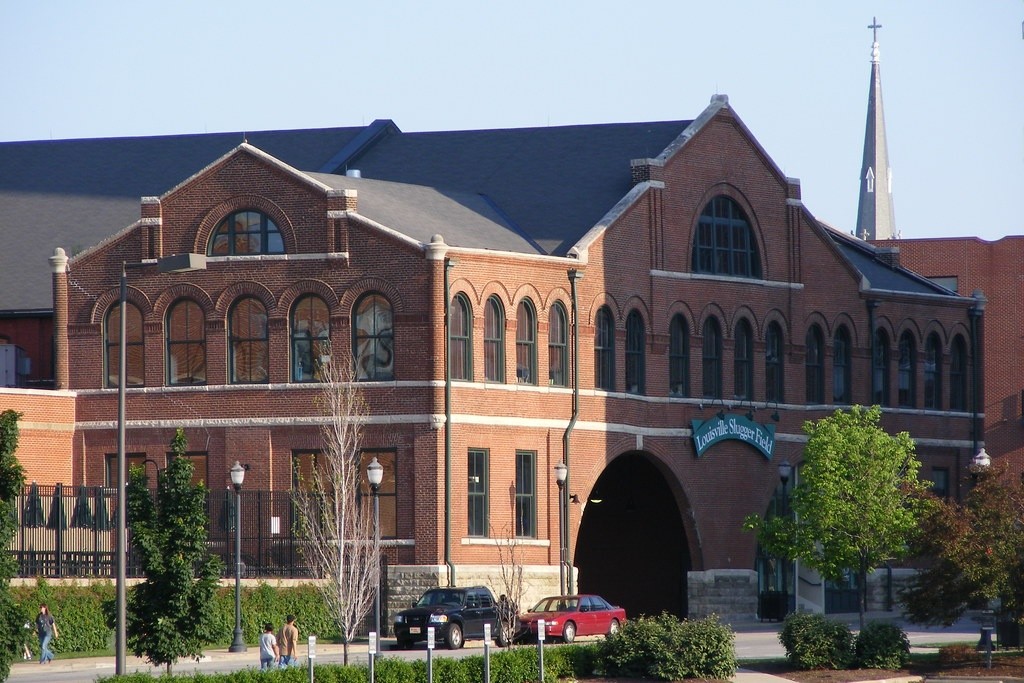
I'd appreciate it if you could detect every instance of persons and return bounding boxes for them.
[259,623,279,669]
[22,619,32,660]
[276,614,299,668]
[35,604,58,664]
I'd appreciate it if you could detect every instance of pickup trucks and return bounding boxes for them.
[394,586,521,651]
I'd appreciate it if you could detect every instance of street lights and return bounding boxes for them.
[115,251,208,675]
[976,447,997,650]
[229,461,247,653]
[554,457,568,610]
[365,455,383,661]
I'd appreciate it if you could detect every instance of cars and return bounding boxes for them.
[518,593,627,644]
